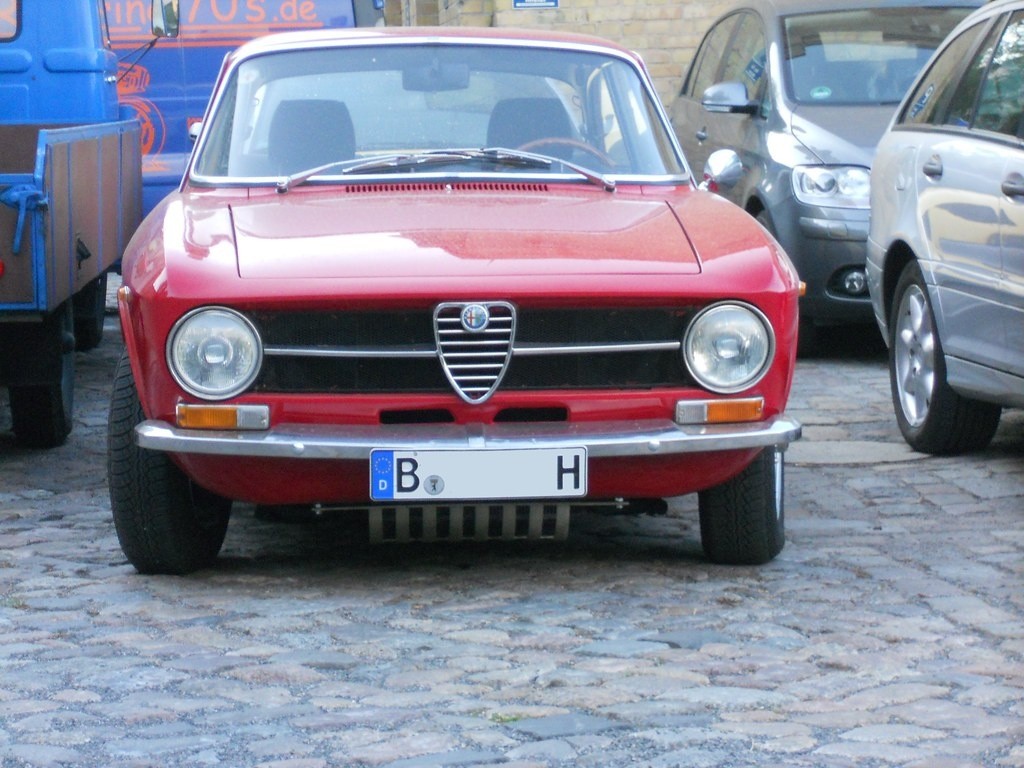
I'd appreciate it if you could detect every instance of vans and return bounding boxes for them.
[0,1,383,234]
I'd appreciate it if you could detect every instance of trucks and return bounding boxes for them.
[0,0,182,442]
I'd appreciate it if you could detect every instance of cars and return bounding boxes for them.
[864,0,1024,453]
[105,24,808,570]
[665,1,992,361]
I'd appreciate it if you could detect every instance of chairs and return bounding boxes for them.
[486,97,576,161]
[266,97,356,168]
